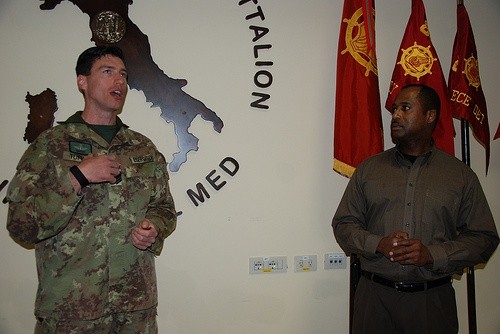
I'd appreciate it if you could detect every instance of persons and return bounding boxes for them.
[6,44,177,334]
[331,83,500,334]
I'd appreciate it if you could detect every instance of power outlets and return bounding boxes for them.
[249,256,287,273]
[325,253,347,269]
[294,255,318,273]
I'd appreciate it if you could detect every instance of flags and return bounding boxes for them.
[332,0,384,179]
[447,4,490,175]
[385,0,456,156]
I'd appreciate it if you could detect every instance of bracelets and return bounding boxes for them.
[69,165,90,187]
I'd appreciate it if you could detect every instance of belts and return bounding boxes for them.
[361,271,452,293]
